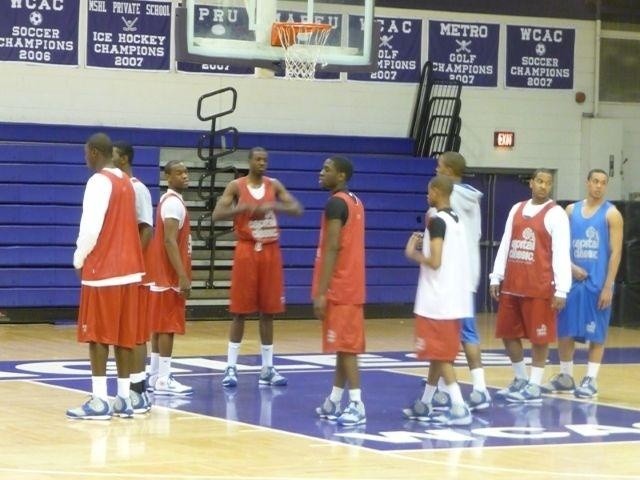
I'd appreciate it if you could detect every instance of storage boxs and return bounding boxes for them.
[609,284,640,328]
[616,238,640,284]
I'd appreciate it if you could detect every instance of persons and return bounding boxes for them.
[112,139,154,413]
[146,160,192,395]
[66,132,147,420]
[211,146,304,386]
[401,175,473,425]
[489,169,573,403]
[430,152,492,410]
[543,169,624,400]
[312,156,366,427]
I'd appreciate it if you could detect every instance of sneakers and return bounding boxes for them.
[223,386,238,400]
[401,390,492,426]
[258,367,288,386]
[496,373,599,402]
[66,373,191,418]
[338,400,366,426]
[315,397,342,420]
[499,403,598,416]
[222,366,237,387]
[260,387,288,400]
